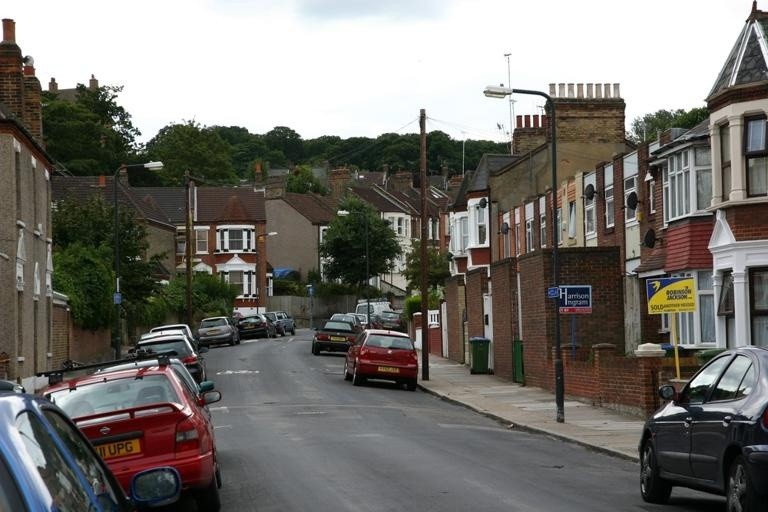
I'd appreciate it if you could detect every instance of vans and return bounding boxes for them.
[353,297,394,318]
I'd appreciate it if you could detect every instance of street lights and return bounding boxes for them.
[338,209,371,330]
[502,52,513,153]
[255,229,278,318]
[483,83,568,424]
[112,157,167,361]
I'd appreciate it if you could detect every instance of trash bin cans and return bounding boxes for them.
[695,348,727,367]
[513,340,523,383]
[469,337,491,374]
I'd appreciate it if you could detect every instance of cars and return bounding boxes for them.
[635,341,768,510]
[343,328,420,391]
[308,309,409,357]
